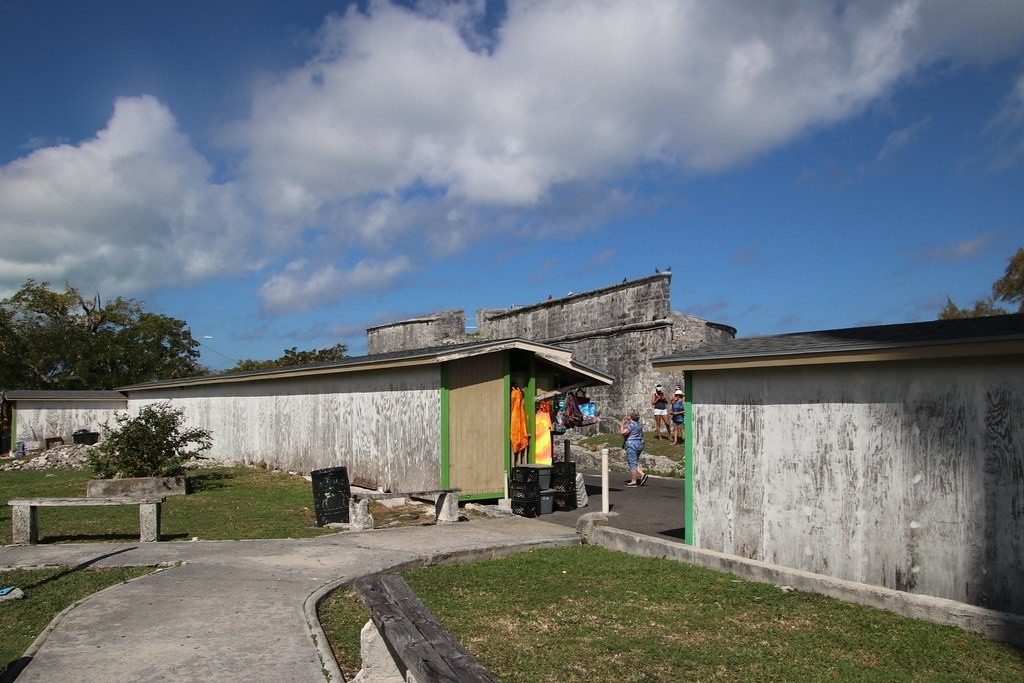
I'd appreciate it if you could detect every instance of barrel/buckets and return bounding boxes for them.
[72,433,99,444]
[310,466,352,526]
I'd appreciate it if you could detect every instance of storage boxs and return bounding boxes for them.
[511,462,588,518]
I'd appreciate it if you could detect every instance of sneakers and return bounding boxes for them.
[640,474,648,486]
[624,483,638,488]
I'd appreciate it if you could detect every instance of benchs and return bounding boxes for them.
[8,496,166,545]
[355,571,501,683]
[349,488,462,529]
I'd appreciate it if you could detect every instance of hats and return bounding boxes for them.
[673,386,685,394]
[655,386,664,392]
[549,294,552,296]
[568,291,573,296]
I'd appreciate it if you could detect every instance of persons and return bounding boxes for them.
[652,385,685,446]
[620,412,648,487]
